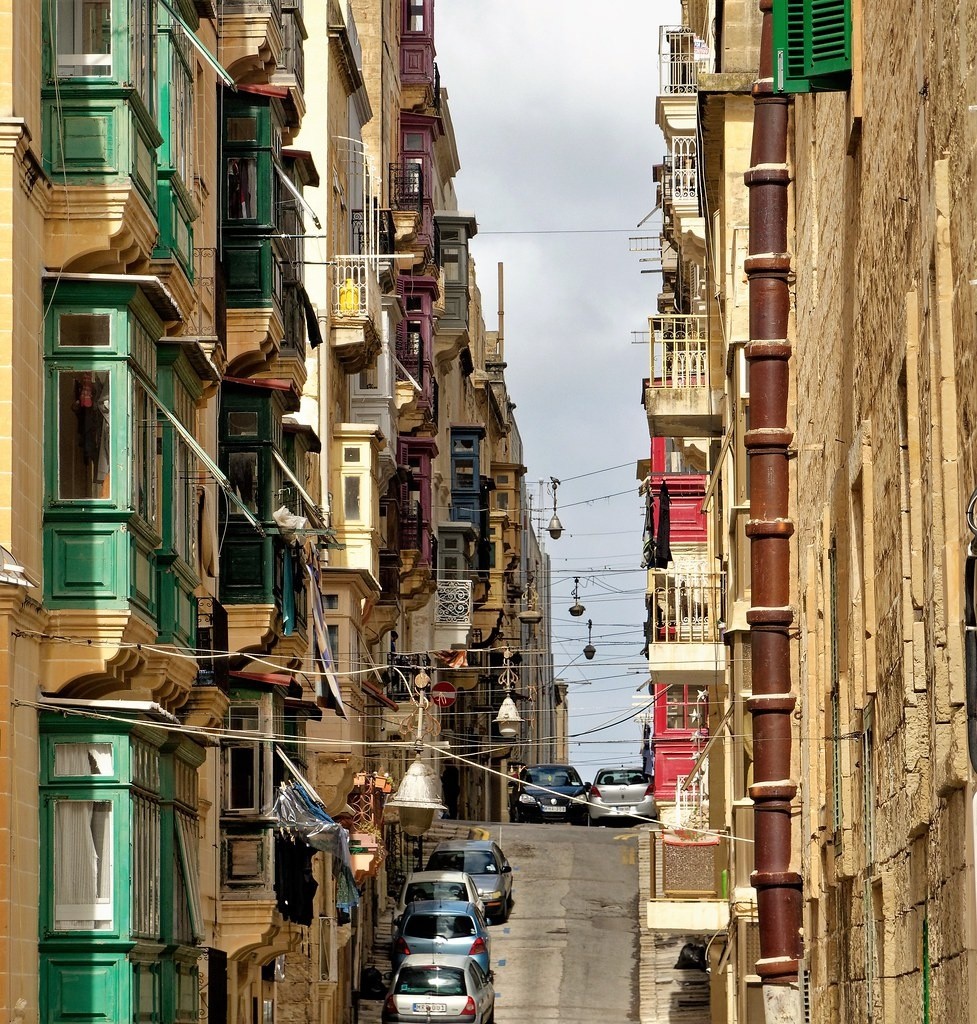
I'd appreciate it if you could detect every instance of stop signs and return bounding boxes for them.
[431,681,457,707]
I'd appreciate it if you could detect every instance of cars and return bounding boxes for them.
[381,953,495,1024]
[507,764,591,826]
[390,870,490,979]
[424,840,514,924]
[588,766,657,825]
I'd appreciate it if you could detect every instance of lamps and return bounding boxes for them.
[568,577,586,617]
[379,667,449,838]
[493,648,525,736]
[582,619,597,660]
[546,475,566,540]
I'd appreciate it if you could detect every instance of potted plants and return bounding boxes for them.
[374,765,388,789]
[382,777,396,793]
